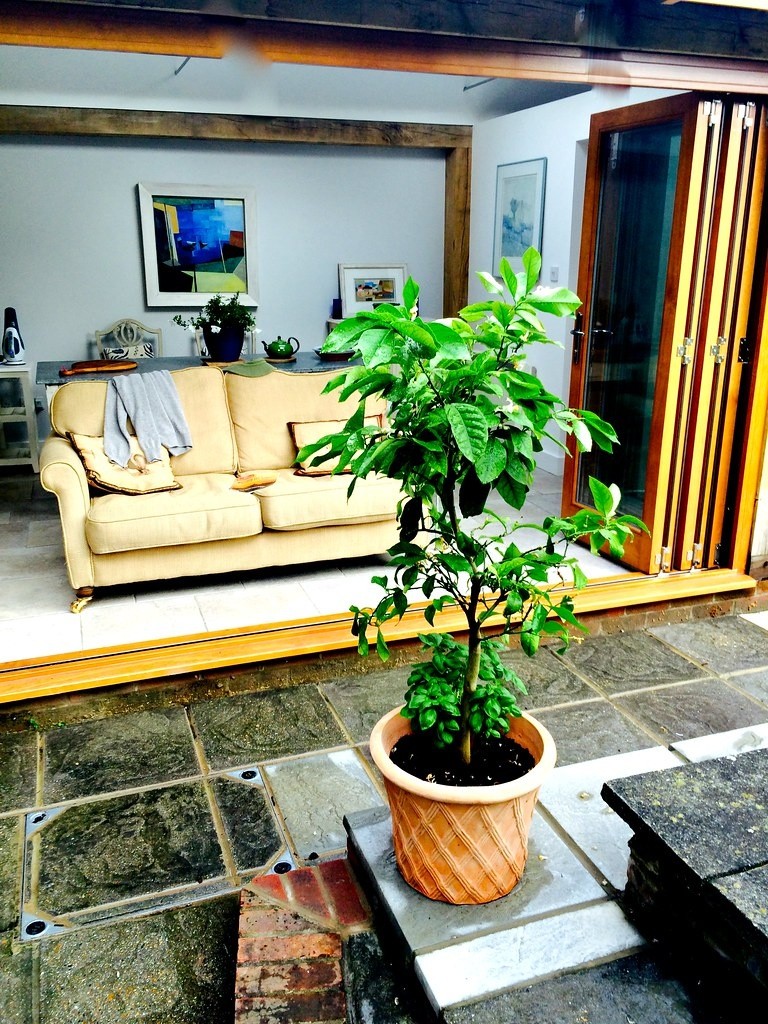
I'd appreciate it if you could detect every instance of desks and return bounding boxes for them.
[36,353,365,414]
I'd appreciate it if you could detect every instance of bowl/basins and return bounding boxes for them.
[314,347,355,362]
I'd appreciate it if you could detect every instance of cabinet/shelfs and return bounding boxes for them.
[0,363,40,473]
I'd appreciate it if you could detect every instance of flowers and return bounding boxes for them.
[172,291,256,333]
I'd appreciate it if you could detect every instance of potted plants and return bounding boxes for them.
[290,246,651,906]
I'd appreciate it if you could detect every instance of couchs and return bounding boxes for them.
[38,358,436,614]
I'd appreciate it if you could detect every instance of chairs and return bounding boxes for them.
[95,318,162,360]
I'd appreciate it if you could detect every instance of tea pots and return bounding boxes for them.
[262,335,300,358]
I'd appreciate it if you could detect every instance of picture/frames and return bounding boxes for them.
[338,263,409,319]
[138,181,259,306]
[491,157,548,280]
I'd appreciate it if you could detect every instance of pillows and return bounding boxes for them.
[66,431,183,495]
[287,415,382,477]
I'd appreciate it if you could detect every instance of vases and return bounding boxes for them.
[202,327,244,361]
